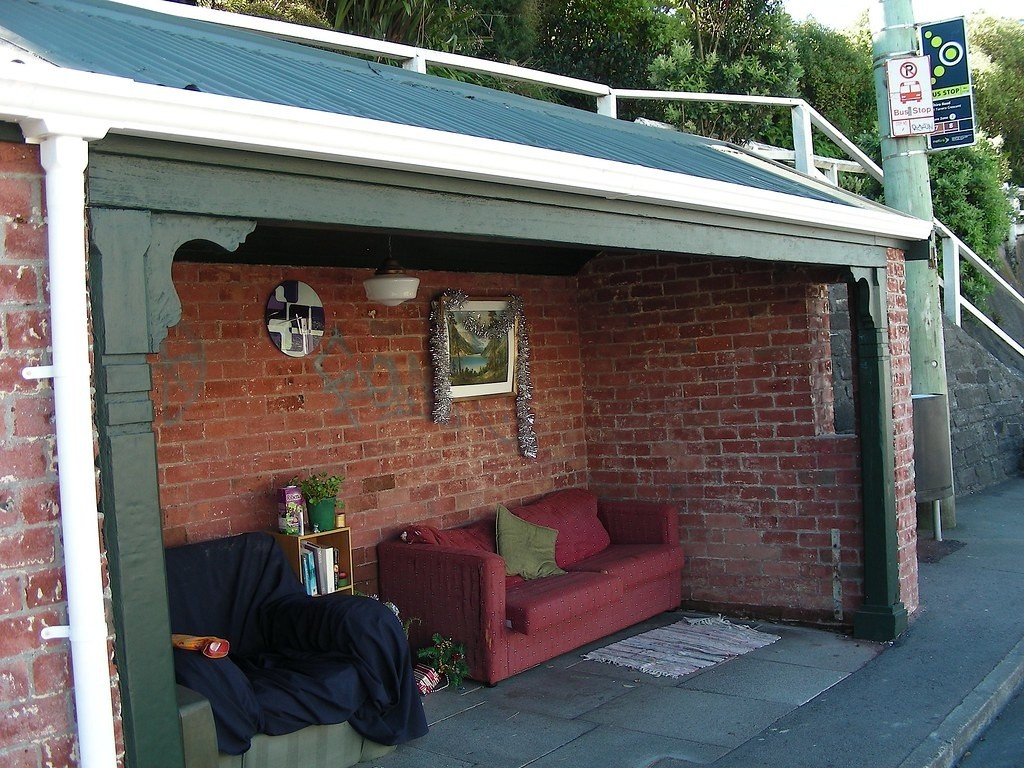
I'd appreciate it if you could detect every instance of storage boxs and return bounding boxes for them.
[413,662,441,697]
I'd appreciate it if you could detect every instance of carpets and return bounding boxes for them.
[579,614,781,680]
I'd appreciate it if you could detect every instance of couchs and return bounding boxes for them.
[375,488,685,690]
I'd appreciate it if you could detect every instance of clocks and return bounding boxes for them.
[265,279,325,357]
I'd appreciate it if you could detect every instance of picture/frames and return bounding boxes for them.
[438,295,519,402]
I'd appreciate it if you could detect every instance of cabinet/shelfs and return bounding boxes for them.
[270,524,353,597]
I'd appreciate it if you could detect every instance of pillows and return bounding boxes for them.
[495,502,568,580]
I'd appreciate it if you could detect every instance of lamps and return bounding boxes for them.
[363,234,420,306]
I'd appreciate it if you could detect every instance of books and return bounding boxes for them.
[300,540,340,597]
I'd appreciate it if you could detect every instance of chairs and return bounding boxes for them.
[165,532,430,768]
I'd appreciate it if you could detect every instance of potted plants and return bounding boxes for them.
[284,472,345,531]
